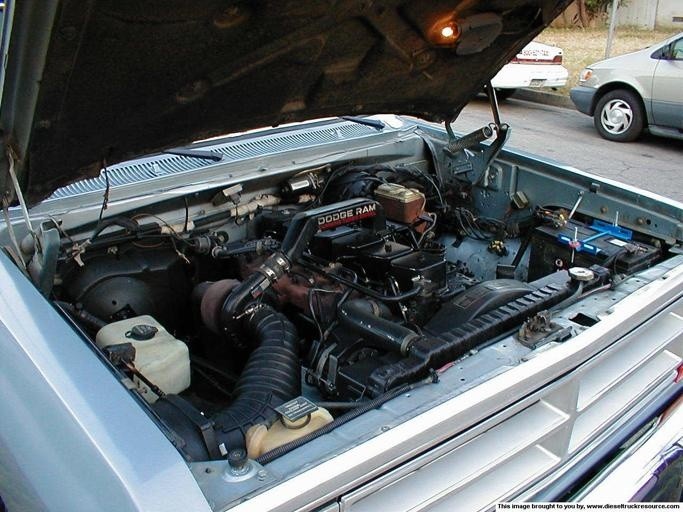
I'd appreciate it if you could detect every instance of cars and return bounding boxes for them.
[0,2,682,511]
[474,42,566,105]
[568,23,683,142]
